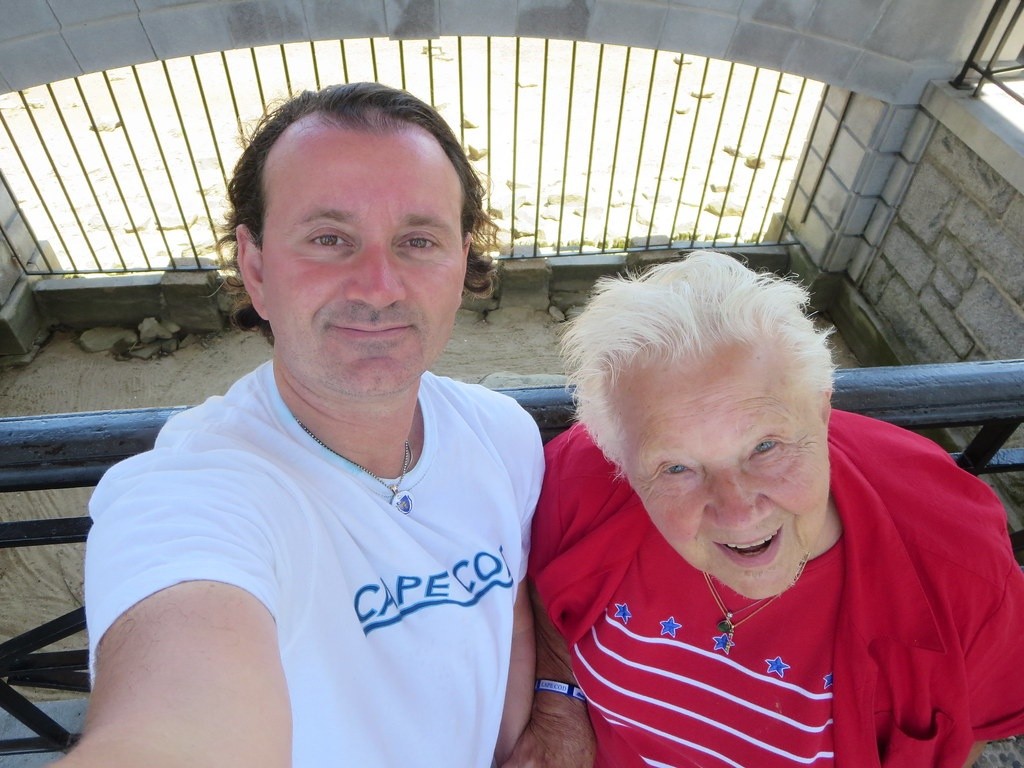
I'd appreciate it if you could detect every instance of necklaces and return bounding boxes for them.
[702,553,810,653]
[291,413,414,516]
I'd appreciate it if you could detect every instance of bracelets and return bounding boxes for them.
[534,678,586,702]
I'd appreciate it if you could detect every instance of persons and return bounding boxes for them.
[39,83,547,768]
[506,250,1023,768]
